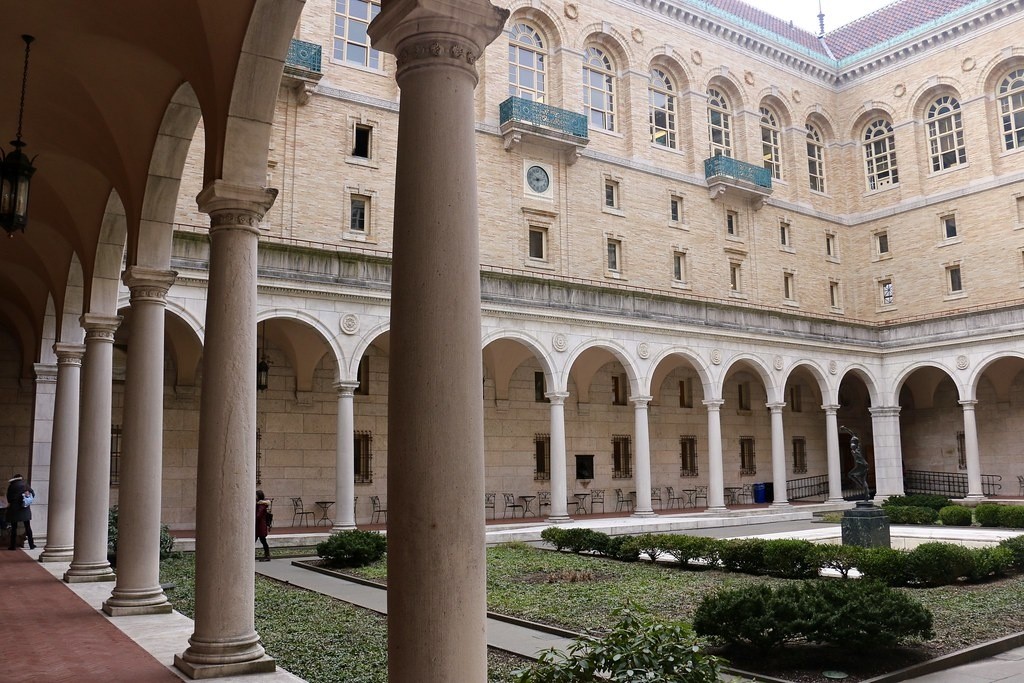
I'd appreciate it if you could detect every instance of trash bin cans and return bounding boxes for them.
[753,482,774,503]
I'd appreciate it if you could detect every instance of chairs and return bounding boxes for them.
[567,497,579,514]
[665,486,684,510]
[538,491,551,518]
[485,493,496,520]
[737,484,754,505]
[651,487,662,511]
[724,495,730,507]
[501,493,524,519]
[265,497,276,532]
[591,490,605,514]
[1016,475,1024,496]
[290,497,316,528]
[354,497,358,527]
[368,495,387,525]
[613,488,632,513]
[694,486,707,508]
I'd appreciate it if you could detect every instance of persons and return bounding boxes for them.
[256,490,271,562]
[0,474,36,550]
[840,426,870,501]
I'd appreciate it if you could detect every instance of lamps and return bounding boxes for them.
[0,34,41,237]
[257,320,268,390]
[766,395,771,412]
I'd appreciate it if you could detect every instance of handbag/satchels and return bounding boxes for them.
[22,491,33,507]
[267,513,273,532]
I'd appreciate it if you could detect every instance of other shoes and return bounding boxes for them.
[260,556,270,562]
[8,547,16,550]
[31,545,36,549]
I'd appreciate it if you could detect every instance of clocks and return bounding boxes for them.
[527,166,549,193]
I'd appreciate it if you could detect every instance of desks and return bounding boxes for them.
[724,487,742,506]
[519,495,535,519]
[574,494,590,514]
[682,490,697,509]
[628,492,636,512]
[315,501,336,527]
